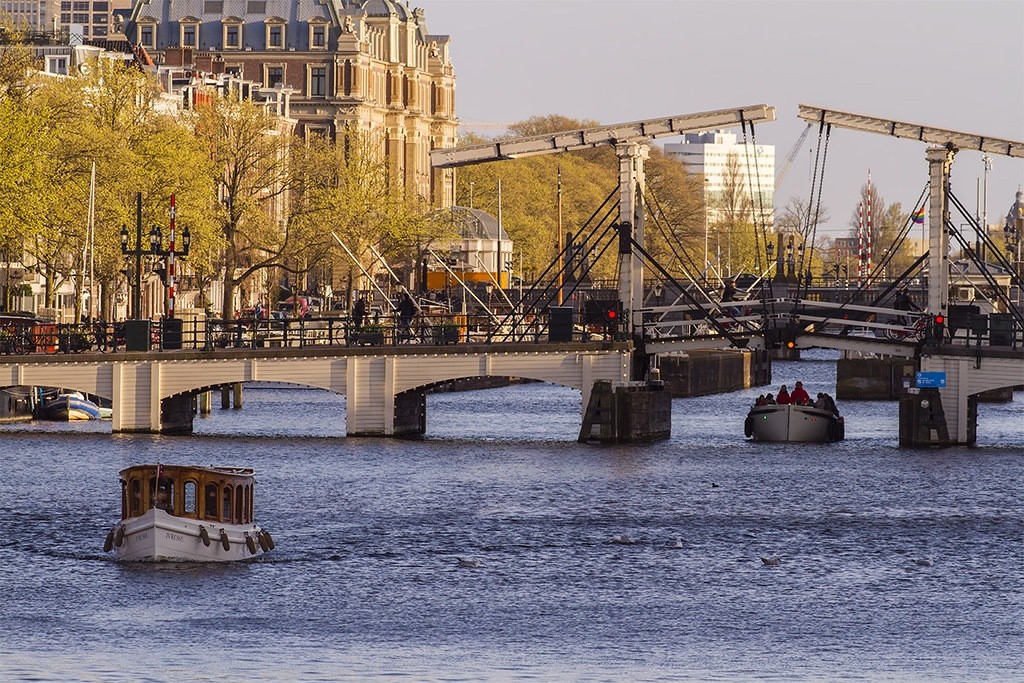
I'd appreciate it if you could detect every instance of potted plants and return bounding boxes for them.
[59,328,93,353]
[432,319,460,345]
[0,326,17,356]
[358,324,384,346]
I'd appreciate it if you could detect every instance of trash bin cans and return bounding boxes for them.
[164,319,182,350]
[548,307,572,342]
[35,321,56,352]
[989,313,1012,346]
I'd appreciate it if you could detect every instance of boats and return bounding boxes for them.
[42,394,100,421]
[106,462,270,562]
[748,404,831,442]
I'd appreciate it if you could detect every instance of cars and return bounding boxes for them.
[237,309,288,327]
[333,301,381,317]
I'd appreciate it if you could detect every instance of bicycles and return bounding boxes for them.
[336,314,368,345]
[884,310,934,341]
[6,321,121,354]
[713,297,764,331]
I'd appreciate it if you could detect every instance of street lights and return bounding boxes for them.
[154,224,191,320]
[120,191,157,320]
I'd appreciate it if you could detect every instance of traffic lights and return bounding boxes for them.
[935,316,944,341]
[608,309,617,335]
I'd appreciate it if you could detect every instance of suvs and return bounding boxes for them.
[275,297,324,318]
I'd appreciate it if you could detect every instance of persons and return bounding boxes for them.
[350,288,447,346]
[256,302,263,330]
[893,288,925,338]
[797,269,813,287]
[755,380,838,414]
[81,314,136,352]
[723,281,751,328]
